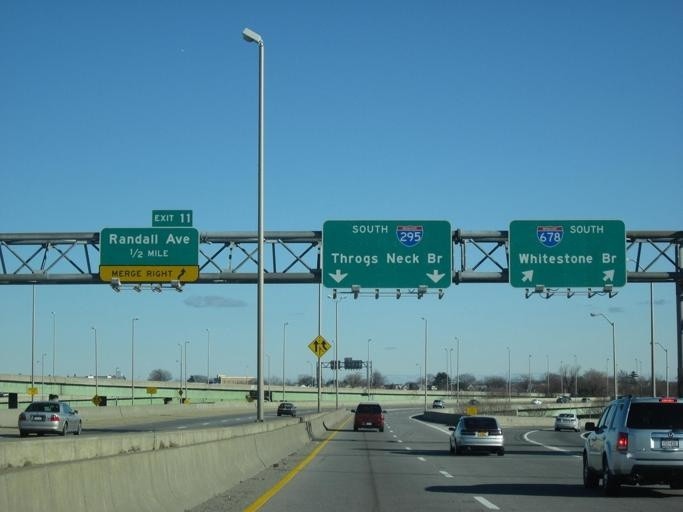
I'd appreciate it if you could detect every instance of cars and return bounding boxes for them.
[17,400,82,437]
[583,398,591,402]
[277,402,296,417]
[467,400,480,406]
[529,400,543,405]
[447,416,504,456]
[553,414,579,432]
[555,397,571,404]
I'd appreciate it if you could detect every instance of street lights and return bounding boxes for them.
[89,325,98,406]
[98,226,198,283]
[505,345,512,409]
[366,337,372,401]
[416,317,460,412]
[558,359,563,394]
[206,327,210,386]
[527,353,530,397]
[649,342,668,398]
[589,311,618,403]
[321,216,452,290]
[184,339,190,400]
[48,311,55,375]
[176,342,182,405]
[39,352,48,399]
[329,291,346,412]
[239,26,266,423]
[572,354,577,393]
[605,356,608,396]
[545,354,549,398]
[129,317,138,406]
[506,217,627,289]
[281,319,287,402]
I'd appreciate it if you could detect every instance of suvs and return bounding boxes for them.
[580,395,682,495]
[350,403,388,434]
